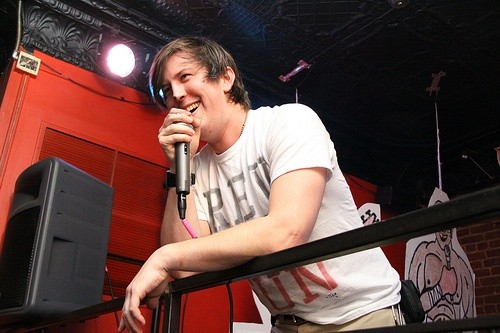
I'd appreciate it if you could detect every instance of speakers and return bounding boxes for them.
[0,157,113,324]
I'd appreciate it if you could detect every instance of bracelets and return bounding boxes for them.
[165,170,196,189]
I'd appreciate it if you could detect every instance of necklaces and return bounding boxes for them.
[210,112,249,155]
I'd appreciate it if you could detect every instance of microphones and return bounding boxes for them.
[172,120,195,218]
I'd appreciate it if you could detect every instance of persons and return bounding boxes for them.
[118,35,425,333]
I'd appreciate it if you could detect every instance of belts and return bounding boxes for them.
[271,314,306,326]
